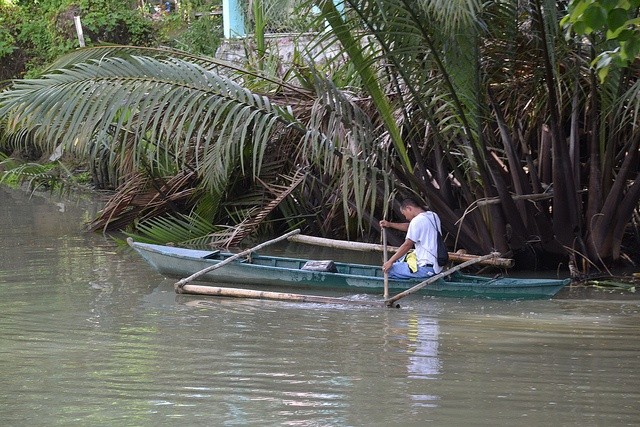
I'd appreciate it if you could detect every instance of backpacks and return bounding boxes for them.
[421,212,449,267]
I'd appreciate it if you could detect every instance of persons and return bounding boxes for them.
[379,198,445,279]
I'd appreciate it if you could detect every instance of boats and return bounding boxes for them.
[126,236,572,300]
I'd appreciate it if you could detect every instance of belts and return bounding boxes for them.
[421,264,435,268]
